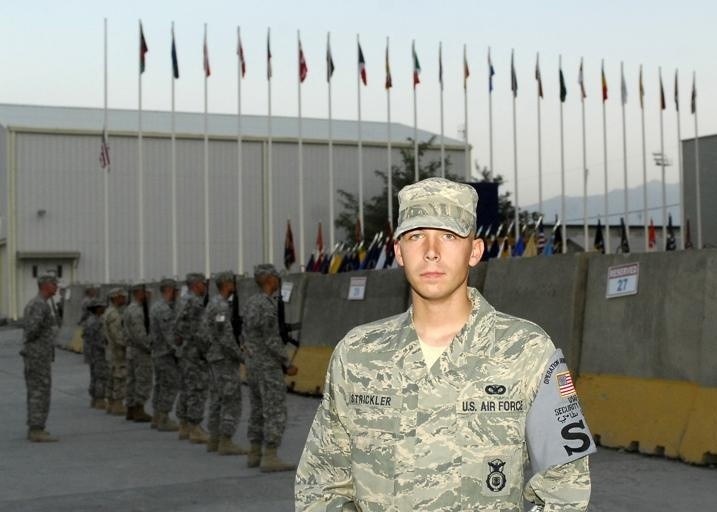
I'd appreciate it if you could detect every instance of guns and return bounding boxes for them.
[126,284,131,305]
[203,279,211,307]
[142,284,150,334]
[231,275,244,345]
[276,276,302,348]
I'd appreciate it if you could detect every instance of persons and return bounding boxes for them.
[236,265,294,472]
[19,276,59,442]
[81,270,248,455]
[294,179,597,511]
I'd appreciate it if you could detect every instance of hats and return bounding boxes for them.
[158,278,181,291]
[214,271,240,281]
[87,297,106,307]
[393,176,479,241]
[184,273,209,283]
[107,286,129,298]
[252,262,280,278]
[37,274,61,285]
[132,282,153,293]
[84,284,101,290]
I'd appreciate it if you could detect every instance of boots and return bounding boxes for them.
[178,418,189,440]
[90,396,95,407]
[95,398,108,409]
[158,411,179,430]
[111,399,127,414]
[246,440,262,468]
[125,403,133,420]
[150,408,158,428]
[217,434,250,455]
[132,402,153,422]
[26,423,58,442]
[260,445,297,471]
[189,422,210,443]
[207,430,218,452]
[105,398,111,413]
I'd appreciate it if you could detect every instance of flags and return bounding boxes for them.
[620,216,630,253]
[646,218,656,245]
[475,217,563,261]
[236,28,308,82]
[666,214,676,252]
[305,220,397,273]
[685,219,693,249]
[439,51,518,96]
[534,63,608,102]
[620,66,696,113]
[326,39,421,88]
[99,128,111,171]
[594,217,605,251]
[139,23,211,78]
[283,219,295,269]
